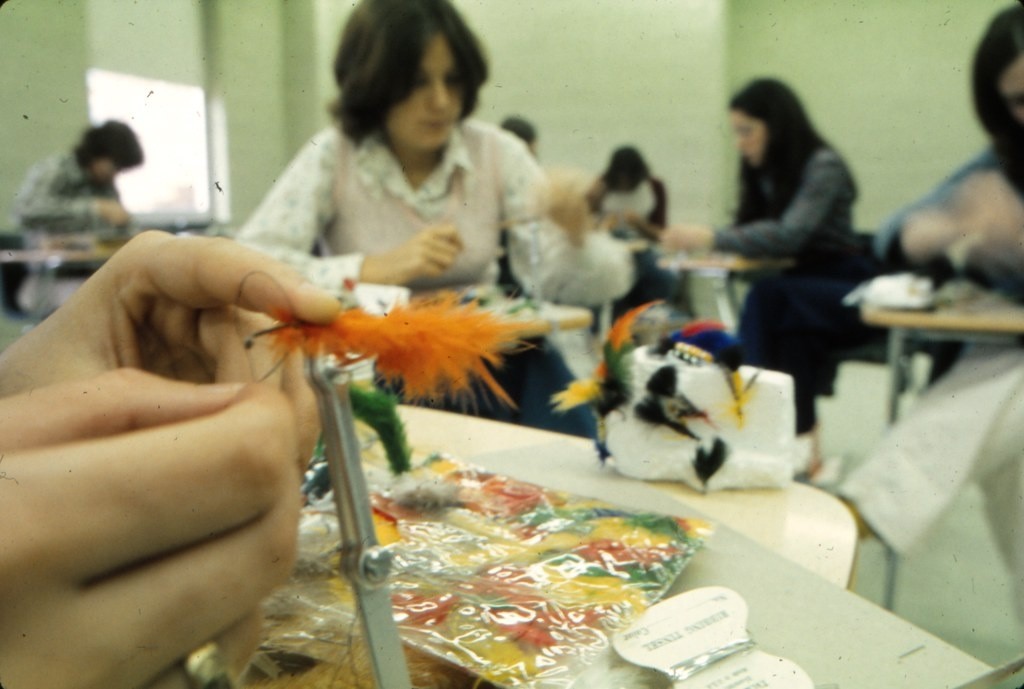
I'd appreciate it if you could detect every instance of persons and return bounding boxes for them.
[874,0,1024,305]
[659,77,858,436]
[496,118,666,338]
[232,0,636,443]
[11,122,142,277]
[0,231,341,689]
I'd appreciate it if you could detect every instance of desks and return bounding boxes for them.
[310,403,864,689]
[653,248,766,340]
[856,274,1024,425]
